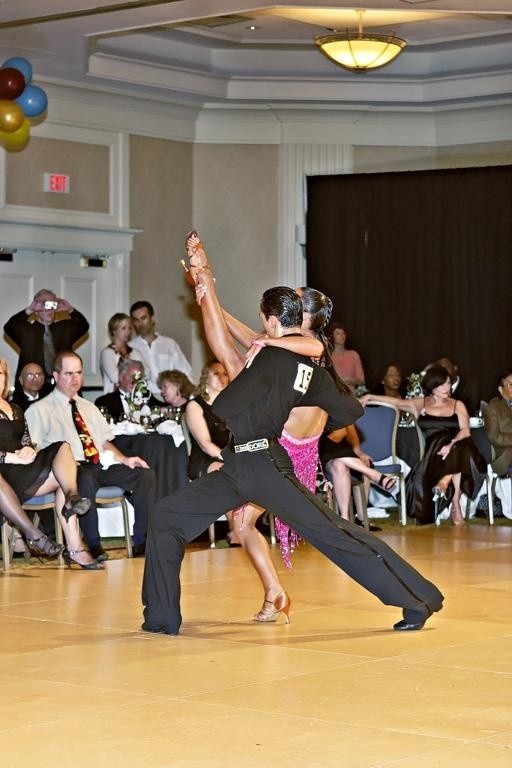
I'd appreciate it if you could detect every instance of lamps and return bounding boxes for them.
[314,9,407,72]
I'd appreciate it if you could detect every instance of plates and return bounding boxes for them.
[470,424,483,428]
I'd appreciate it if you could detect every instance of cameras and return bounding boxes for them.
[43,300,58,311]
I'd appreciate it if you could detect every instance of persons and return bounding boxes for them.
[142,286,444,636]
[1,289,244,570]
[317,328,512,534]
[178,230,335,625]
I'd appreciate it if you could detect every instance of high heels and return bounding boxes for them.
[62,493,92,523]
[180,230,212,286]
[27,534,64,558]
[62,546,103,569]
[255,591,291,623]
[432,486,446,502]
[451,506,465,525]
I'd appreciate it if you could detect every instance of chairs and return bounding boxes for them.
[326,399,510,533]
[1,414,275,570]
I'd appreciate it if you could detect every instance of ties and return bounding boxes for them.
[70,399,99,465]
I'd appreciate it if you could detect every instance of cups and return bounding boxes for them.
[469,416,483,426]
[97,404,183,428]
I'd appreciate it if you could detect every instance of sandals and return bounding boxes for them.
[378,474,396,491]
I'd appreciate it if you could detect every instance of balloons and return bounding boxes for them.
[1,57,49,151]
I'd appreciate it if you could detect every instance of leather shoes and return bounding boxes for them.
[142,621,179,634]
[355,516,383,531]
[394,603,441,629]
